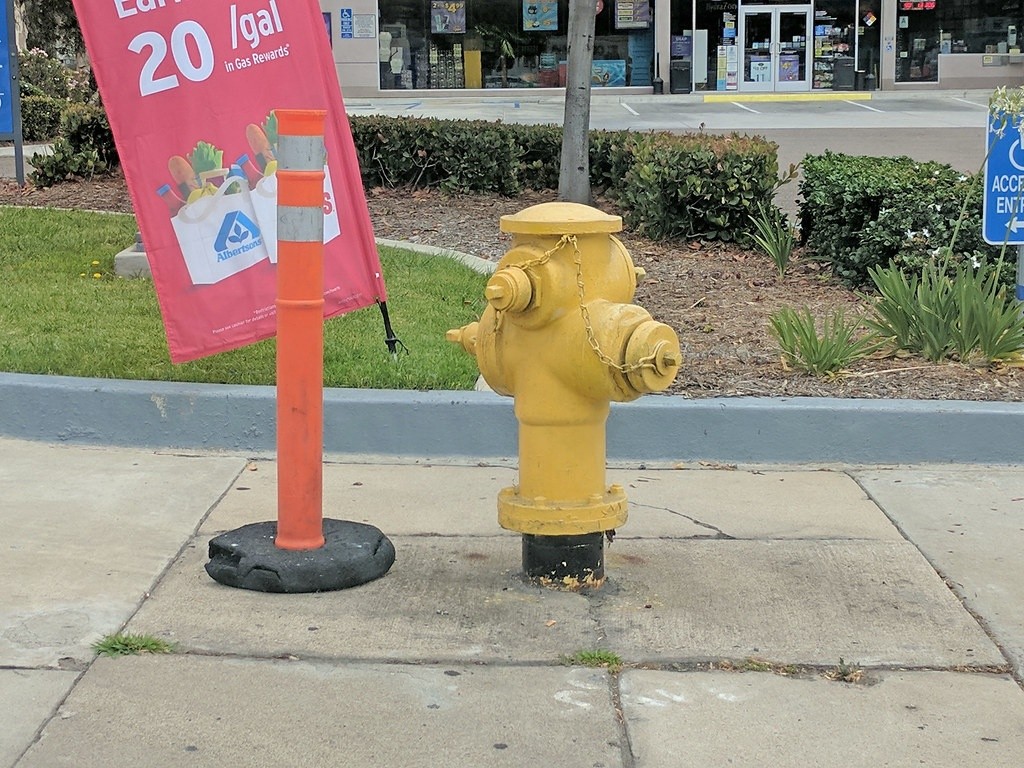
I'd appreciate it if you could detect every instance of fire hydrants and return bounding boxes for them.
[444,200,685,589]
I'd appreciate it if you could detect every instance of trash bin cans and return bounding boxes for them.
[834,58,855,91]
[671,61,690,94]
